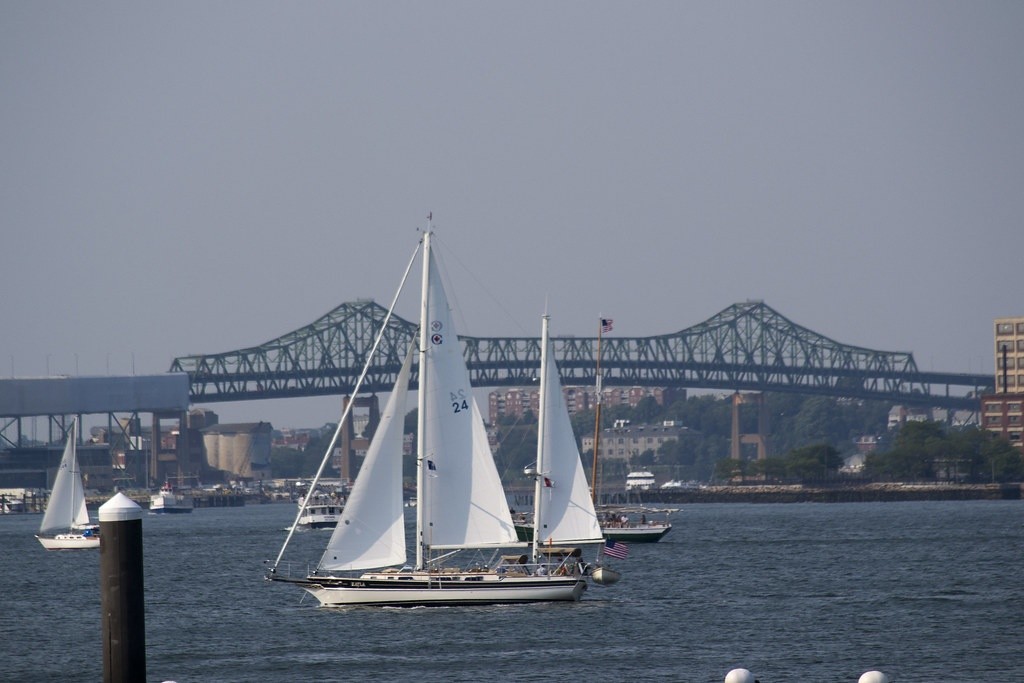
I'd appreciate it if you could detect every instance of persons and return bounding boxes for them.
[621,514,629,528]
[640,514,648,525]
[535,562,548,576]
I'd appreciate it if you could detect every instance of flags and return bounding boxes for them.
[543,476,558,489]
[603,537,628,560]
[600,316,613,334]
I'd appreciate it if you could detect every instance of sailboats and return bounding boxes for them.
[261,206,606,608]
[35,415,101,550]
[508,315,673,543]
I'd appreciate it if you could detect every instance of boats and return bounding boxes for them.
[591,566,621,586]
[294,493,348,528]
[148,481,194,512]
